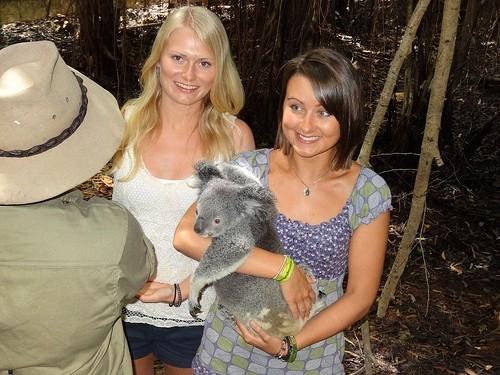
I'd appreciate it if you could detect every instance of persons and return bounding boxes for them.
[111,6,255,375]
[172,48,393,375]
[0,41,158,375]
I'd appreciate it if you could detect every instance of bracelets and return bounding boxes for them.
[274,335,297,363]
[168,283,182,307]
[273,254,294,282]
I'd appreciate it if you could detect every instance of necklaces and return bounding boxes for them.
[290,147,339,196]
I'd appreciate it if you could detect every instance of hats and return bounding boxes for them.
[0,40,127,205]
